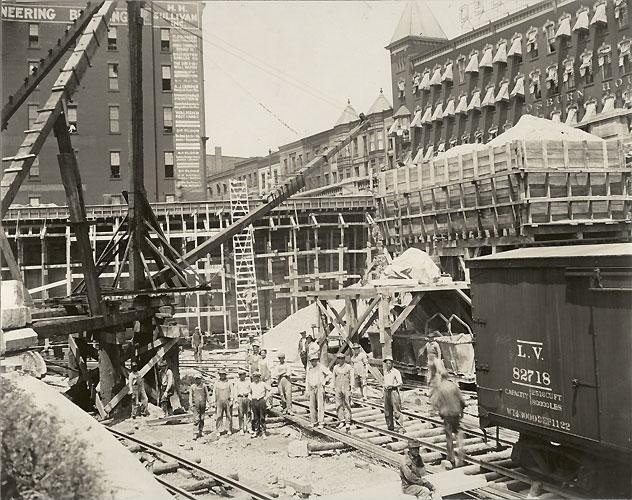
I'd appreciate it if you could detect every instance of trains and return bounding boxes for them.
[463,241,632,499]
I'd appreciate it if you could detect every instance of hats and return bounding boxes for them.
[158,361,165,367]
[132,361,137,367]
[406,440,421,448]
[195,373,202,380]
[426,333,434,337]
[277,353,285,359]
[218,368,262,378]
[300,330,313,340]
[249,334,267,354]
[308,354,318,361]
[336,353,346,357]
[383,356,394,362]
[351,343,361,349]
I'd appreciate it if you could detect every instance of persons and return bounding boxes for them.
[187,325,408,441]
[398,439,444,500]
[427,370,466,466]
[127,360,149,420]
[416,333,443,388]
[157,358,176,421]
[242,286,254,320]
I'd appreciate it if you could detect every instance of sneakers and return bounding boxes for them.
[282,411,295,416]
[192,431,267,440]
[308,423,350,430]
